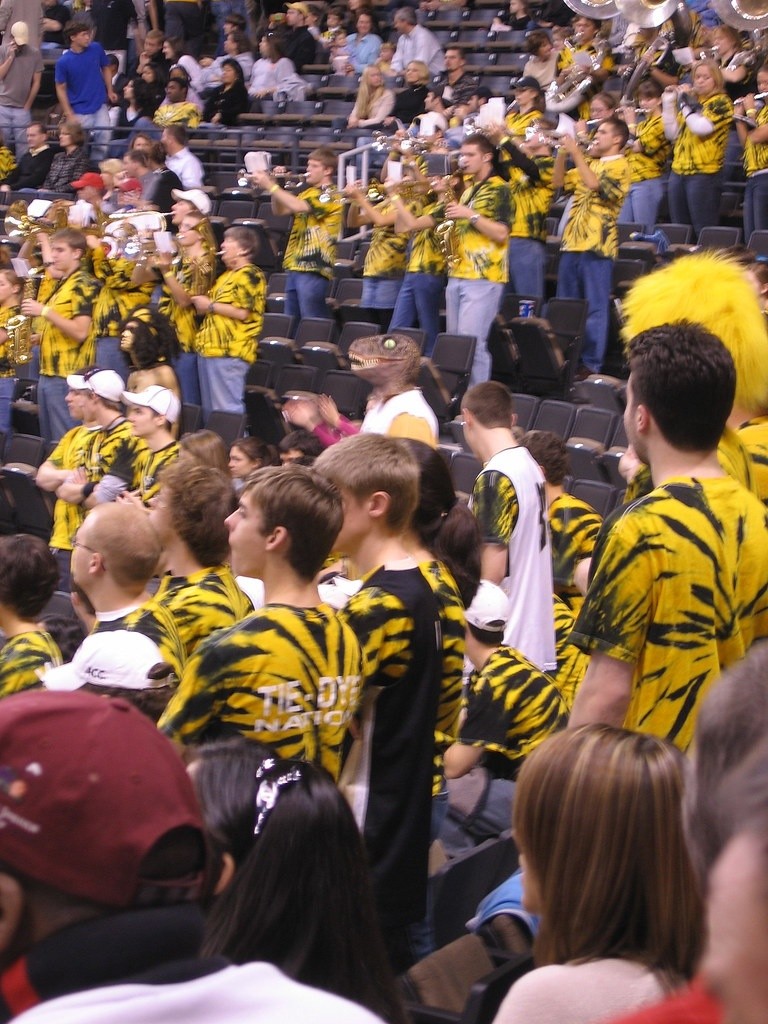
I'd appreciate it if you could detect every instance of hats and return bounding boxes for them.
[284,2,307,18]
[172,188,214,214]
[121,386,181,424]
[11,22,29,45]
[65,367,126,404]
[43,628,173,691]
[114,178,142,193]
[1,688,213,909]
[71,172,103,190]
[464,579,510,632]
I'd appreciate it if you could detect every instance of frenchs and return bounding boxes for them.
[543,0,767,114]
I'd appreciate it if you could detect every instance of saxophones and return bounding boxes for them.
[433,165,469,271]
[4,261,54,366]
[189,214,228,298]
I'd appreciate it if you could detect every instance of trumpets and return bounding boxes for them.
[2,197,187,262]
[236,116,605,204]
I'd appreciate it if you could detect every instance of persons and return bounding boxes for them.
[0,0,578,234]
[0,188,267,458]
[0,250,768,1024]
[245,0,768,391]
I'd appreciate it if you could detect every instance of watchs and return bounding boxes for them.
[469,213,480,226]
[208,301,215,313]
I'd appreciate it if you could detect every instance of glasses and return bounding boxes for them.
[69,535,105,571]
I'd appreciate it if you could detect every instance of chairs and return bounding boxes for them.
[0,0,768,1024]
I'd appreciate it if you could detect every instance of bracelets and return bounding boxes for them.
[41,306,49,317]
[269,184,279,194]
[163,270,174,279]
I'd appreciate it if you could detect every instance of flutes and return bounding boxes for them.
[504,83,768,125]
